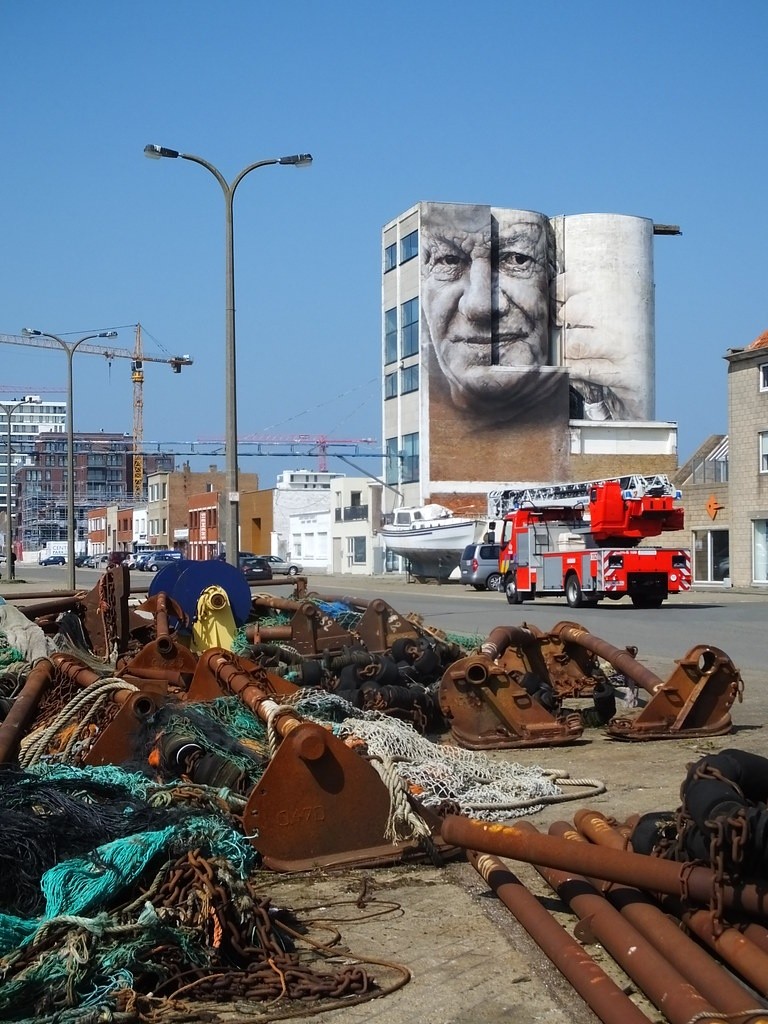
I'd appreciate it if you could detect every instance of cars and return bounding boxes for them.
[716,557,730,580]
[82,550,156,570]
[147,555,176,572]
[239,557,274,580]
[214,551,257,563]
[253,555,304,575]
[73,555,91,568]
[0,555,8,563]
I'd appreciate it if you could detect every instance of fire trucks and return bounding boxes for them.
[486,472,695,611]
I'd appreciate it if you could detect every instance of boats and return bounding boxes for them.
[381,503,491,578]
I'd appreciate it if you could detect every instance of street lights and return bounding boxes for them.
[0,399,42,581]
[22,327,118,590]
[142,144,317,564]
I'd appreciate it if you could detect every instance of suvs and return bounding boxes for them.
[459,542,504,593]
[39,555,66,566]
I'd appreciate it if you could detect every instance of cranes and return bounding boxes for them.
[195,432,380,473]
[0,332,194,496]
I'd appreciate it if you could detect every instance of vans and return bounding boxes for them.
[139,550,184,572]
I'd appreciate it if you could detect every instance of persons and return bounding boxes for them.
[11,548,17,580]
